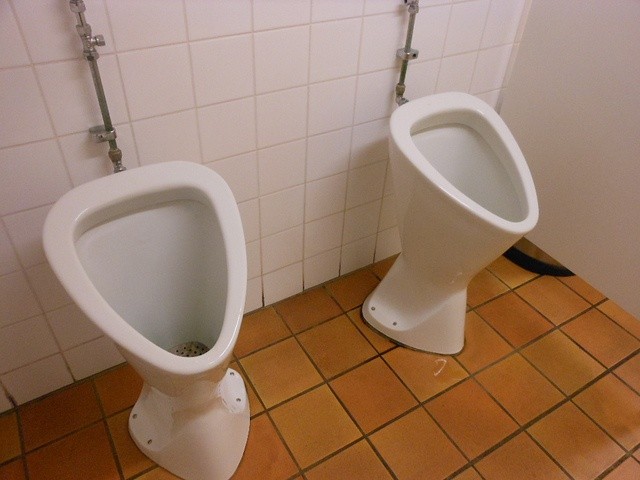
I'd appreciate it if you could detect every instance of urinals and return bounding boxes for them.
[362,93,540,356]
[43,160,250,479]
